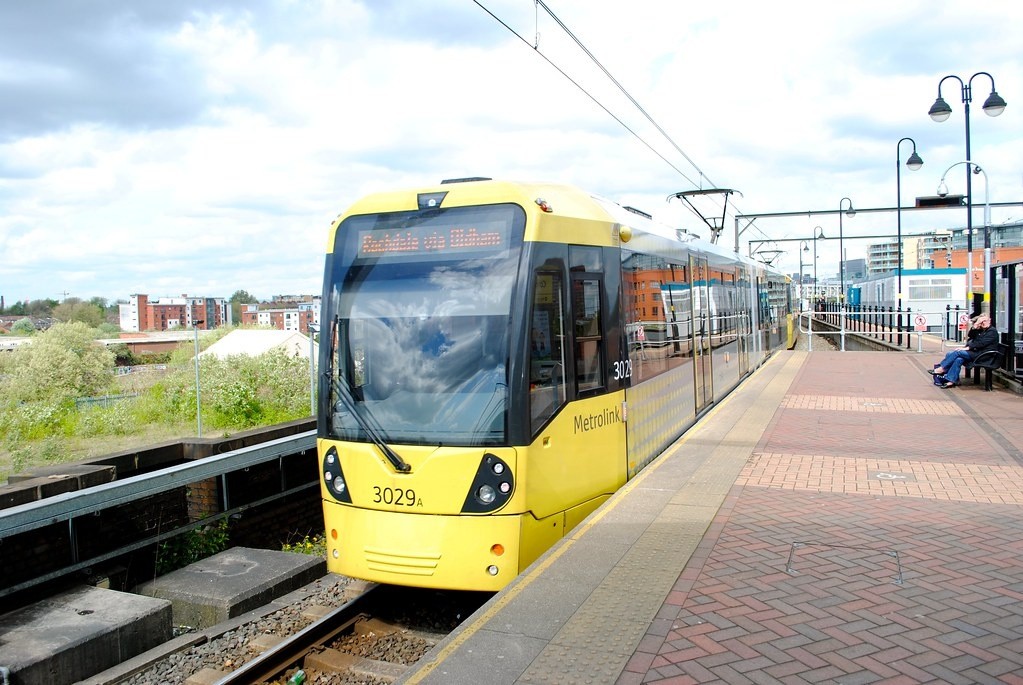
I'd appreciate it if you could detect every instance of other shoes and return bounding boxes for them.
[928,370,945,376]
[943,383,955,388]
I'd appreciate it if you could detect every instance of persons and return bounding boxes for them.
[817,295,826,321]
[927,312,1000,389]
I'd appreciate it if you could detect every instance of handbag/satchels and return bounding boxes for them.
[934,364,961,385]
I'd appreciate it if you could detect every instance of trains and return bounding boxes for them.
[303,172,800,594]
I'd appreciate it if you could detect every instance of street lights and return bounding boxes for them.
[799,240,811,314]
[307,323,321,416]
[838,197,857,309]
[192,319,205,437]
[813,225,825,317]
[896,137,925,344]
[927,70,1007,321]
[936,161,992,317]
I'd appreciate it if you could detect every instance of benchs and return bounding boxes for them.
[966,342,1008,391]
